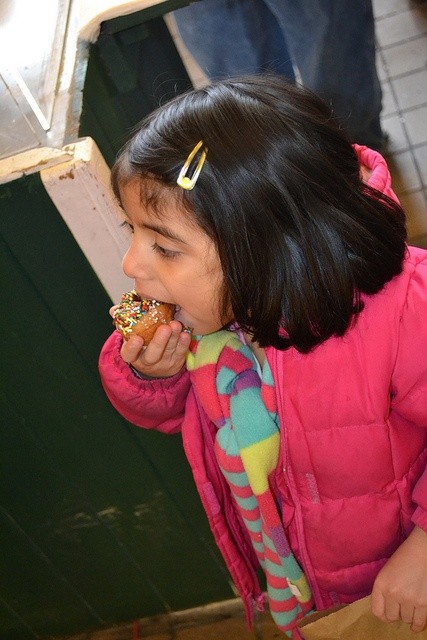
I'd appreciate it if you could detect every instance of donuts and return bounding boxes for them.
[112,290,177,348]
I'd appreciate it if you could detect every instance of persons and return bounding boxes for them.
[96,78,426,635]
[172,0,384,153]
